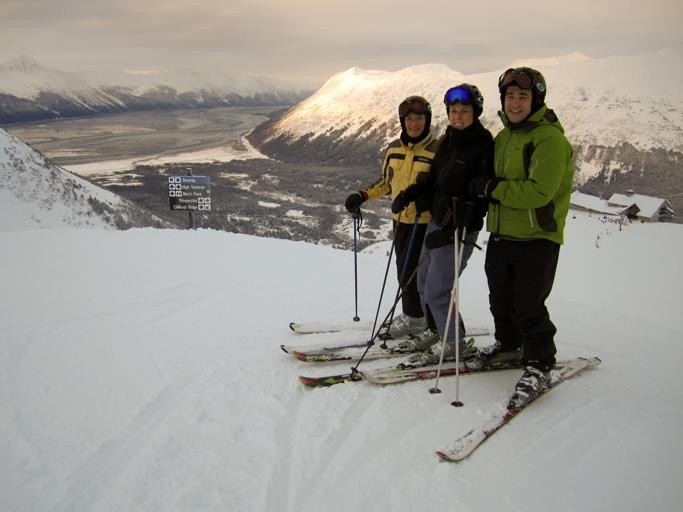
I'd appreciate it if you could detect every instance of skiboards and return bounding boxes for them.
[291,337,490,387]
[358,356,601,386]
[280,322,490,356]
[280,322,600,462]
[435,356,593,462]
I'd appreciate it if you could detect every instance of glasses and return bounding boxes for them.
[445,87,472,105]
[399,101,426,117]
[499,70,536,89]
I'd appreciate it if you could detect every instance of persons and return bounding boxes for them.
[392,85,494,366]
[475,67,575,405]
[344,96,442,337]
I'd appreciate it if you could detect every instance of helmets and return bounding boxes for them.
[498,67,546,113]
[445,84,483,118]
[399,96,431,143]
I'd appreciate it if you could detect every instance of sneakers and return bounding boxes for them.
[515,367,552,396]
[388,313,466,361]
[476,342,520,363]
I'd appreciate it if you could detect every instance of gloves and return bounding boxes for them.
[345,191,367,212]
[425,230,455,249]
[468,175,504,203]
[392,191,412,213]
